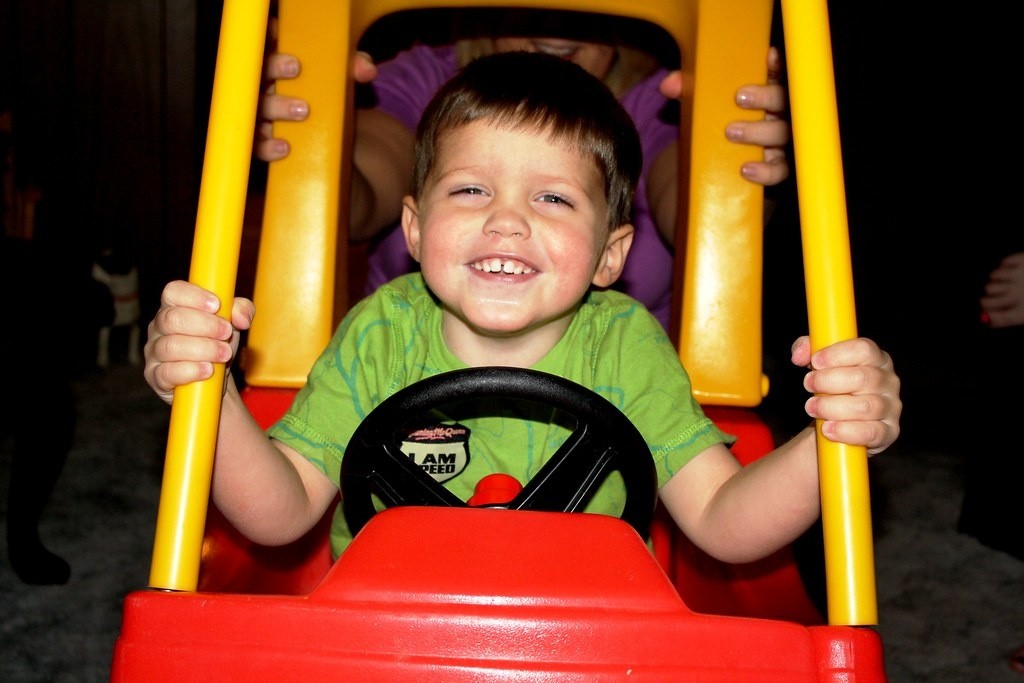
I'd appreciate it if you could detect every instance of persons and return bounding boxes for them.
[902,0,1024,560]
[0,0,190,588]
[251,7,792,339]
[144,50,903,569]
